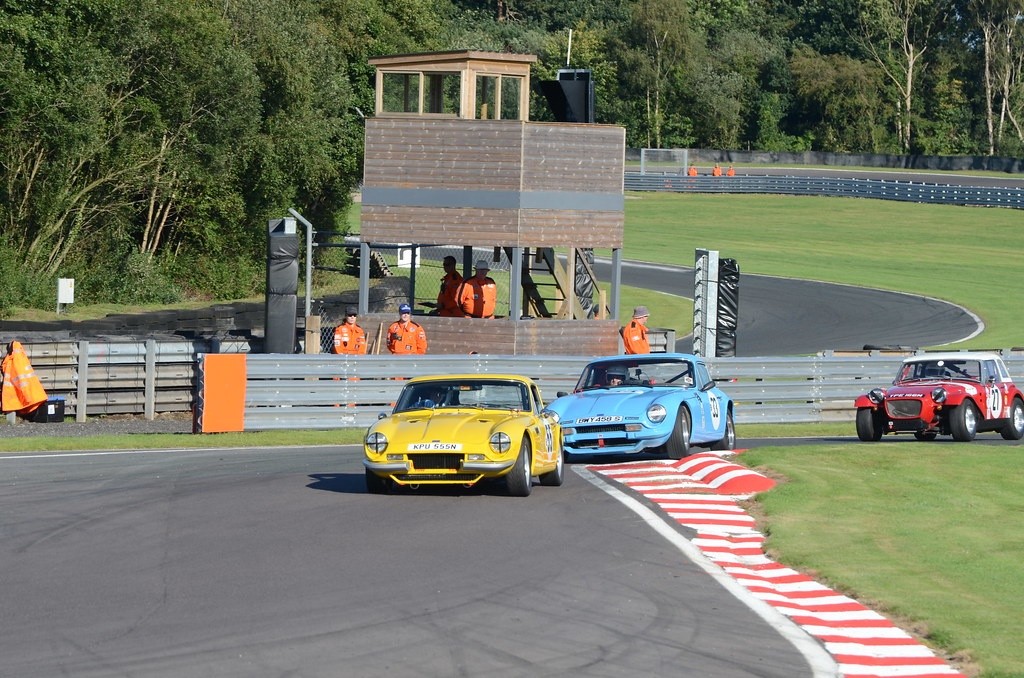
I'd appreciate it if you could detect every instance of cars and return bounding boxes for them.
[543,353,737,462]
[363,375,564,496]
[855,351,1024,442]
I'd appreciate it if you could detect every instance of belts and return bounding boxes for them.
[468,314,491,318]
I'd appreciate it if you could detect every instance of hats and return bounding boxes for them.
[399,304,411,312]
[473,260,491,271]
[633,306,650,319]
[345,306,357,315]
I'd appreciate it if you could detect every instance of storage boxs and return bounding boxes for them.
[23,400,64,423]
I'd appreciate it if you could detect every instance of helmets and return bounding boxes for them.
[605,364,630,383]
[925,362,945,378]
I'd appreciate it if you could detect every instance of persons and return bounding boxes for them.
[712,163,722,176]
[623,306,650,355]
[408,388,446,408]
[332,305,366,407]
[387,304,428,406]
[605,364,630,387]
[687,163,697,188]
[593,303,599,320]
[455,260,496,319]
[726,164,735,176]
[925,363,945,378]
[432,256,465,317]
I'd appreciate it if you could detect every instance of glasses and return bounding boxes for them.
[443,262,450,266]
[348,314,357,317]
[402,312,409,314]
